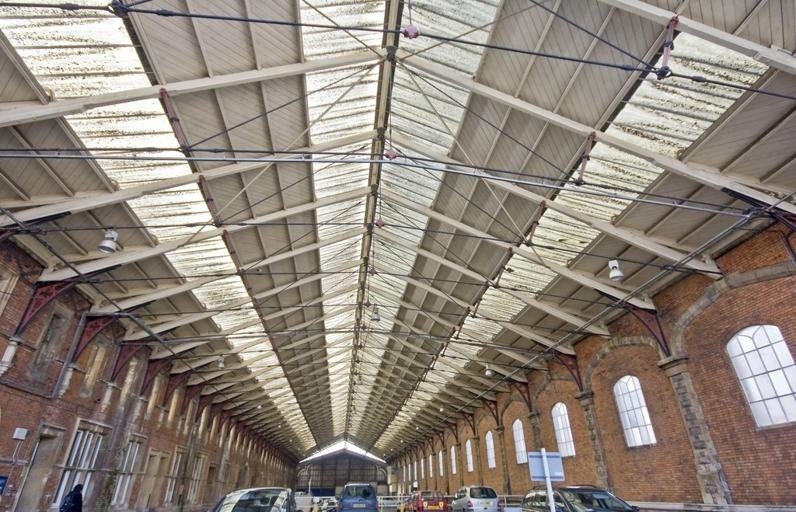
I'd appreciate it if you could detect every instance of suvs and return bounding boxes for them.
[521,483,640,512]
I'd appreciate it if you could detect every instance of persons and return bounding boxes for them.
[59,483,83,511]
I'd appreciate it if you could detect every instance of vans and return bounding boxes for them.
[450,485,501,512]
[335,482,378,512]
[404,490,447,512]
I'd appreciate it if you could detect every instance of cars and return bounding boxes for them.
[211,487,295,512]
[376,496,397,509]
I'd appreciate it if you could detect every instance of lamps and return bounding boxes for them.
[98,230,118,253]
[609,259,623,280]
